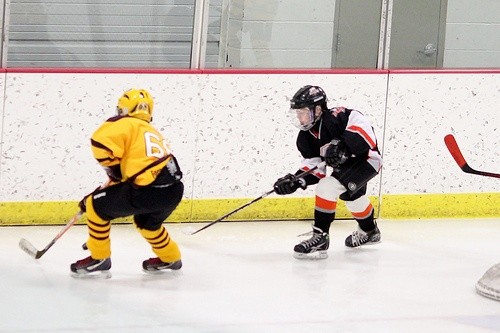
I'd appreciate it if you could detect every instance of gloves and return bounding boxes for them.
[274,174,305,195]
[325,143,347,169]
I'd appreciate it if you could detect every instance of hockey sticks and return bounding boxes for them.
[444,134,500,180]
[18,176,112,260]
[184,160,329,236]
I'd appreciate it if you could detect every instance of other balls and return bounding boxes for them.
[82,242,88,250]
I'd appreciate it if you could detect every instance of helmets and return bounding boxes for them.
[287,85,326,131]
[116,88,153,123]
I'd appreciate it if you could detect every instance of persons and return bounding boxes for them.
[71,87,183,280]
[274,85,384,261]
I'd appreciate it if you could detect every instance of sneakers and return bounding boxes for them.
[143,257,182,273]
[293,224,329,259]
[345,220,381,248]
[69,256,112,278]
